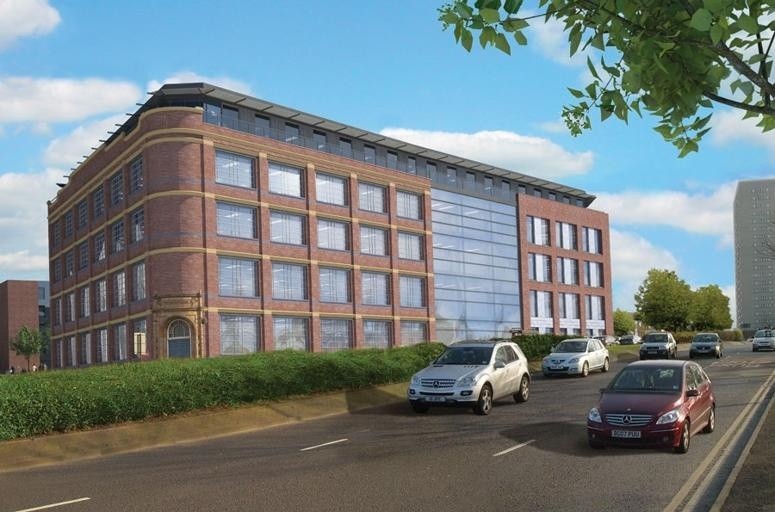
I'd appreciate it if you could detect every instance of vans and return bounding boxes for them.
[590,336,605,342]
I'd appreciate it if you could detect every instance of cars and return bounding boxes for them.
[583,359,716,453]
[541,339,610,377]
[406,339,531,415]
[688,333,723,359]
[751,329,775,352]
[639,332,677,359]
